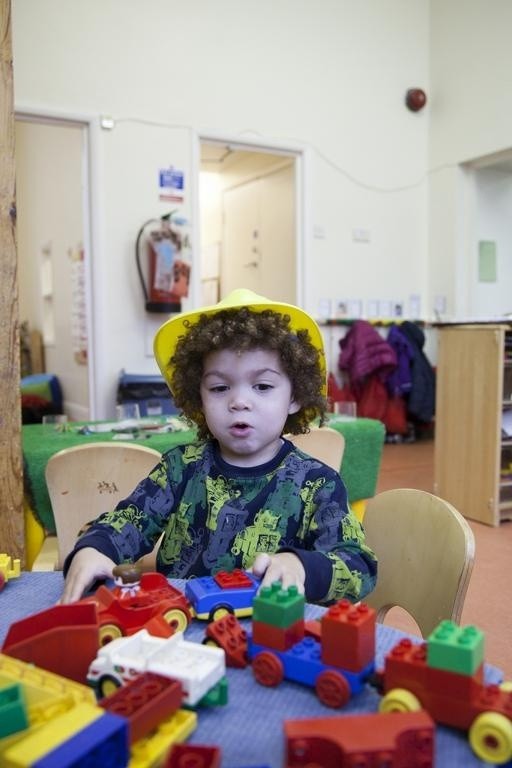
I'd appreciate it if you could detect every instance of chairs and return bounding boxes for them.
[46,439,172,570]
[360,488,476,640]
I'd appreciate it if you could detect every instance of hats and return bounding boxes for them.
[154,289,328,433]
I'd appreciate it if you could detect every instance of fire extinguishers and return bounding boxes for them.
[135,208,182,312]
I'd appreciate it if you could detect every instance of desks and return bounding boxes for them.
[26,412,387,537]
[0,568,511,768]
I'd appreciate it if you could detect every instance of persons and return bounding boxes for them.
[57,286,379,607]
[112,563,142,600]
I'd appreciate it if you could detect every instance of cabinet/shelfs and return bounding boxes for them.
[432,323,512,528]
